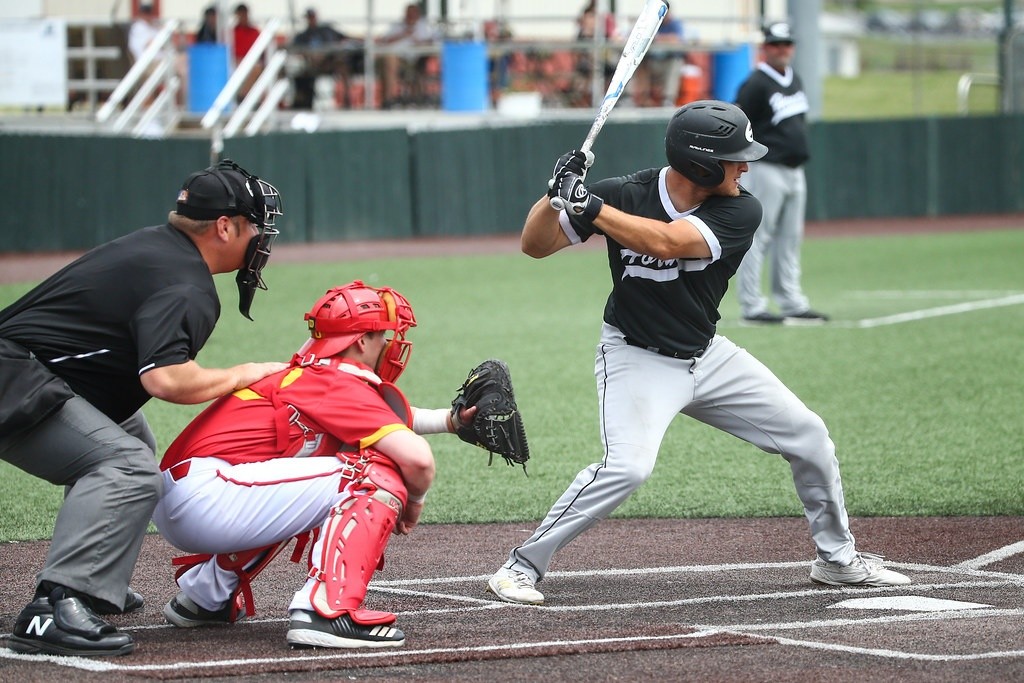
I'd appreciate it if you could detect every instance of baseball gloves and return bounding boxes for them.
[448,359,531,479]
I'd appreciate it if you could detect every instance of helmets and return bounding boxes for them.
[665,100,768,187]
[296,286,389,359]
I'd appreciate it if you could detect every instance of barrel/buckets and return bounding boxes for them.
[440,40,488,111]
[711,42,752,105]
[187,44,229,113]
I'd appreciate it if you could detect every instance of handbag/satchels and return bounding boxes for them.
[0,339,74,437]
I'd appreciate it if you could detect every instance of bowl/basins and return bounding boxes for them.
[497,92,543,111]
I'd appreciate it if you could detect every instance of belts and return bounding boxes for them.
[170,460,191,481]
[626,337,704,359]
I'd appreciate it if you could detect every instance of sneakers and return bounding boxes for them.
[87,586,144,614]
[488,565,545,607]
[161,592,248,627]
[808,550,911,588]
[7,595,134,657]
[287,605,405,649]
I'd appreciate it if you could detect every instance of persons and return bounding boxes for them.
[127,0,689,111]
[153,279,477,648]
[487,98,913,604]
[0,155,284,654]
[736,20,827,323]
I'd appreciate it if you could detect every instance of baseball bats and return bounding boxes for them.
[549,0,671,212]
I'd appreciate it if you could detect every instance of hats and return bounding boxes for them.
[177,164,263,229]
[764,22,795,45]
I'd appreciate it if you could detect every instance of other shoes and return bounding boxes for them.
[784,308,828,326]
[741,314,784,327]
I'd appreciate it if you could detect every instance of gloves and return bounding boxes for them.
[547,172,605,222]
[552,150,597,183]
